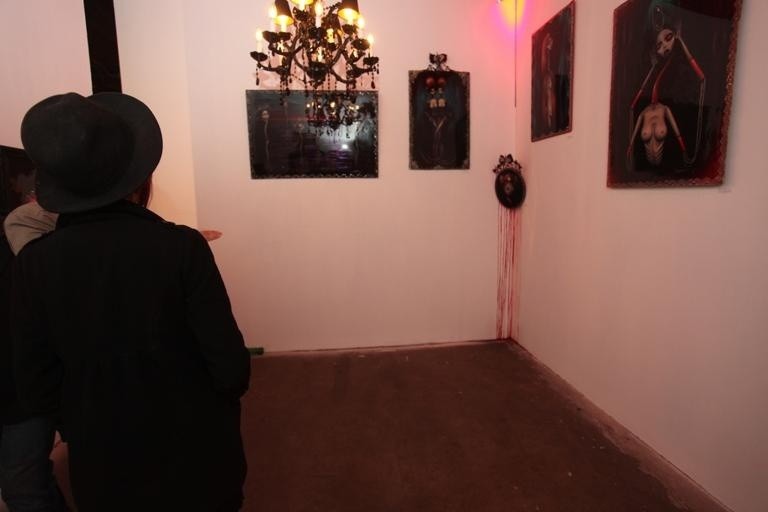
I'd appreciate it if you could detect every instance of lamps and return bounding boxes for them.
[250,0,380,112]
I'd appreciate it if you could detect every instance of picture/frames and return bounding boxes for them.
[529,1,574,143]
[606,1,743,192]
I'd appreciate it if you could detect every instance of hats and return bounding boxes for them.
[20,90,164,214]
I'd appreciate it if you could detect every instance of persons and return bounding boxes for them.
[623,23,707,169]
[0,145,34,270]
